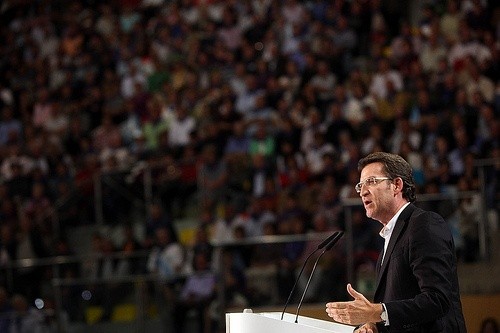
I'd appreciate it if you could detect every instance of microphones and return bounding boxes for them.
[281,231,339,320]
[295,231,344,323]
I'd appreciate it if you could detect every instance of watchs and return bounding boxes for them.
[380,304,386,324]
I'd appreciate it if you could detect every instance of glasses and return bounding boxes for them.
[355,177,394,192]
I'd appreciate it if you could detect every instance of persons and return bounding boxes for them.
[0,0,500,333]
